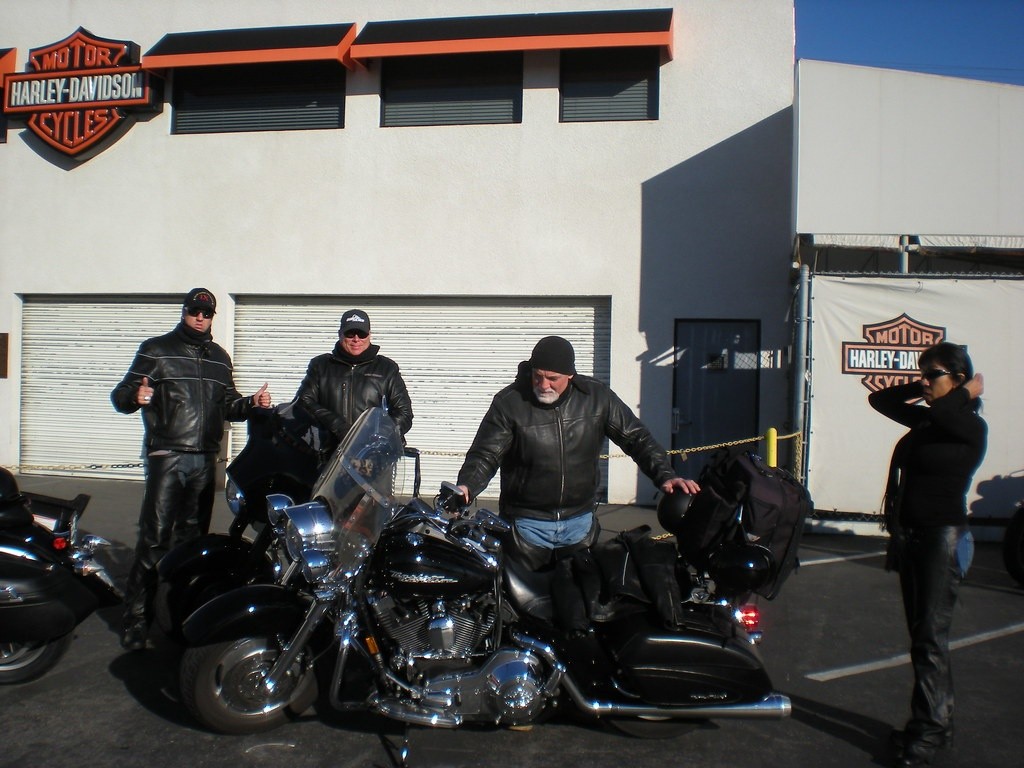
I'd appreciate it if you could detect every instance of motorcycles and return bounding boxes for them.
[141,388,802,767]
[0,465,126,685]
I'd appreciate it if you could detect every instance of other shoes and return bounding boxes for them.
[888,728,914,741]
[898,749,924,766]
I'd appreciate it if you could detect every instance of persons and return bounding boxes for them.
[111,288,271,649]
[292,309,413,527]
[446,336,700,730]
[868,343,988,768]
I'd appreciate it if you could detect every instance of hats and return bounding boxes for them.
[528,336,575,375]
[184,287,217,314]
[339,309,370,334]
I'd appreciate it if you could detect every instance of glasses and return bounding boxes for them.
[187,307,213,319]
[343,329,368,339]
[920,369,952,380]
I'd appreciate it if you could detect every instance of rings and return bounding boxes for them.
[145,396,151,401]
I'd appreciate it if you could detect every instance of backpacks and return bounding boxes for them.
[694,443,815,600]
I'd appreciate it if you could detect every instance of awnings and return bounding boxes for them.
[142,8,673,78]
